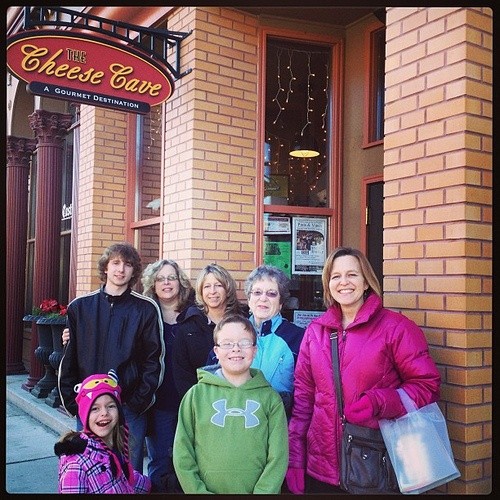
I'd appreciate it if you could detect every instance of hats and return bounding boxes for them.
[74,368,134,485]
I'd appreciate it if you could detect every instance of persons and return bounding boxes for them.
[57,242,168,475]
[61,258,216,495]
[53,366,155,494]
[170,314,290,495]
[298,233,324,251]
[207,264,306,430]
[286,246,443,495]
[194,260,254,330]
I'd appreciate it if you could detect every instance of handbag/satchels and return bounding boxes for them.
[340,420,399,493]
[378,388,461,495]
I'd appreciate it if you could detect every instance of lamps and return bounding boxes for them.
[288,88,320,157]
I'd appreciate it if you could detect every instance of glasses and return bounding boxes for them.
[249,289,279,297]
[155,274,178,282]
[215,339,255,349]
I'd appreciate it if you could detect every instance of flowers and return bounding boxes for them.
[40,299,68,316]
